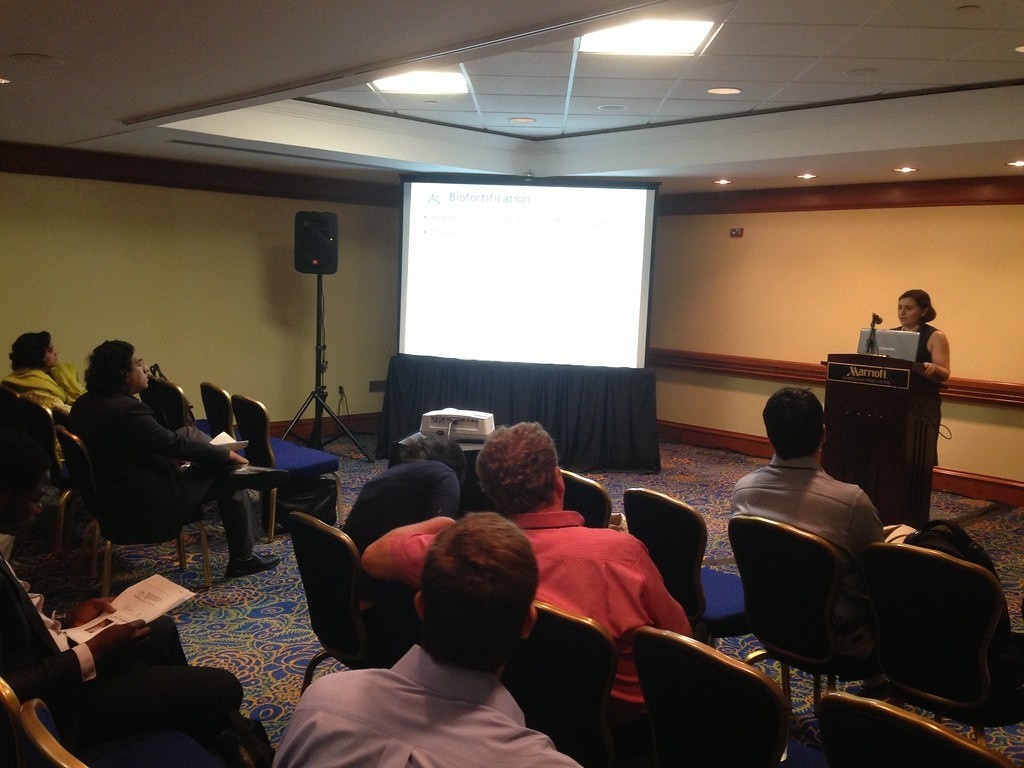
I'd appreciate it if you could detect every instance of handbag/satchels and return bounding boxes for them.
[150,364,197,427]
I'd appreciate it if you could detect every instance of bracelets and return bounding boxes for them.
[934,364,937,370]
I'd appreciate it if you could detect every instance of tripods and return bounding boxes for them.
[280,274,375,463]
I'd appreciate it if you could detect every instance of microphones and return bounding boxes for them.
[873,313,883,324]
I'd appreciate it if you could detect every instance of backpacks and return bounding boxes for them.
[904,518,1013,690]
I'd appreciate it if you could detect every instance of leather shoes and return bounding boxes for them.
[230,465,291,491]
[226,550,280,577]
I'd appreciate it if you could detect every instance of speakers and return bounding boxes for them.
[294,211,338,275]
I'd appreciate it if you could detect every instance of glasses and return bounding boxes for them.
[123,359,146,371]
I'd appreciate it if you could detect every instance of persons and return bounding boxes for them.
[343,421,712,760]
[0,331,83,478]
[65,340,290,578]
[890,289,950,467]
[270,512,584,768]
[0,428,276,768]
[731,386,918,695]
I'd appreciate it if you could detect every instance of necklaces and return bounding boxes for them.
[902,326,919,332]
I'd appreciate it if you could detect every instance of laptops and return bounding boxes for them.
[857,329,920,362]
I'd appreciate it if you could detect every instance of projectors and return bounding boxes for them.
[420,407,495,444]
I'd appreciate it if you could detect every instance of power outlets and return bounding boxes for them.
[369,381,387,391]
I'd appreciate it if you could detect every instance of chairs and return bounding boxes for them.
[562,470,611,529]
[814,691,1012,768]
[55,421,215,593]
[621,485,751,643]
[524,604,639,768]
[288,512,361,700]
[146,375,195,430]
[15,391,66,570]
[200,379,237,462]
[726,515,857,707]
[858,541,1024,747]
[627,627,790,768]
[231,393,346,544]
[20,697,222,768]
